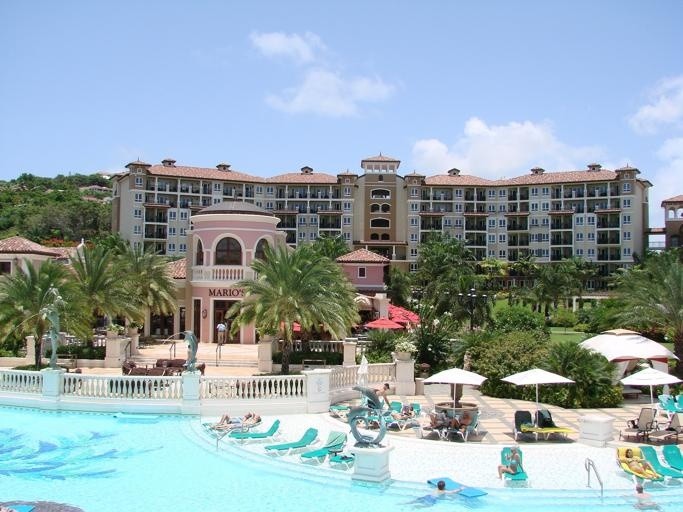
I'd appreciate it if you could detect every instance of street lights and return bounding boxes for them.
[458,288,487,331]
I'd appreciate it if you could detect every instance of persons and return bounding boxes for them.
[635,484,664,512]
[217,319,227,344]
[617,449,656,479]
[430,409,472,433]
[210,412,261,430]
[367,383,393,423]
[497,447,524,480]
[396,481,465,509]
[391,406,415,420]
[182,345,192,367]
[463,351,471,371]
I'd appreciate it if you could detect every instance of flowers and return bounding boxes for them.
[395,341,419,353]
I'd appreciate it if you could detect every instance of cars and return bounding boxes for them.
[95,327,107,335]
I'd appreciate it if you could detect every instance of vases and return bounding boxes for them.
[397,352,411,361]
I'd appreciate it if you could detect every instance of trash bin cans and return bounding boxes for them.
[420,363,431,385]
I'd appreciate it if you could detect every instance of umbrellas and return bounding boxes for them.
[619,367,683,430]
[500,369,576,440]
[421,368,488,415]
[281,304,421,332]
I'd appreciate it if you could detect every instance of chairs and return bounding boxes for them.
[204,412,361,470]
[326,397,481,445]
[507,408,571,443]
[617,394,683,488]
[496,445,530,484]
